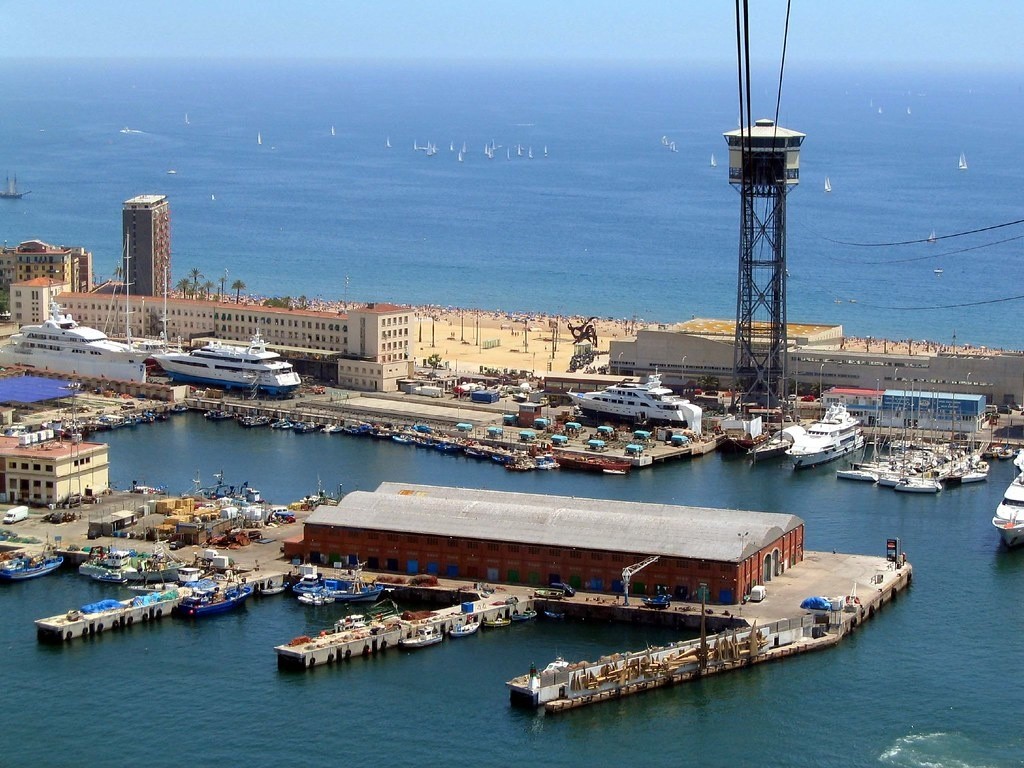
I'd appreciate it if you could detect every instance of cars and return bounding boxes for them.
[801,394,815,402]
[788,394,796,400]
[690,389,718,396]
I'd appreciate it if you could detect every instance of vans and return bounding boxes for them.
[269,506,289,515]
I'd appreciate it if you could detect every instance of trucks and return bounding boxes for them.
[751,585,767,601]
[3,506,29,524]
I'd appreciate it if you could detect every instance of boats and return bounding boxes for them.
[641,595,670,610]
[41,401,189,437]
[0,171,32,199]
[261,560,402,634]
[78,529,228,599]
[0,532,63,582]
[542,647,569,673]
[566,373,690,428]
[399,609,565,649]
[534,588,565,599]
[0,226,301,398]
[177,582,254,618]
[204,410,632,473]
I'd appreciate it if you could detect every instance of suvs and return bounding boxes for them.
[170,541,184,549]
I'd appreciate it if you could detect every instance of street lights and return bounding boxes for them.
[820,363,825,420]
[681,356,687,380]
[618,352,624,374]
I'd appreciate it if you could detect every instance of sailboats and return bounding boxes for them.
[958,152,969,169]
[722,397,1024,544]
[823,175,832,193]
[926,229,936,242]
